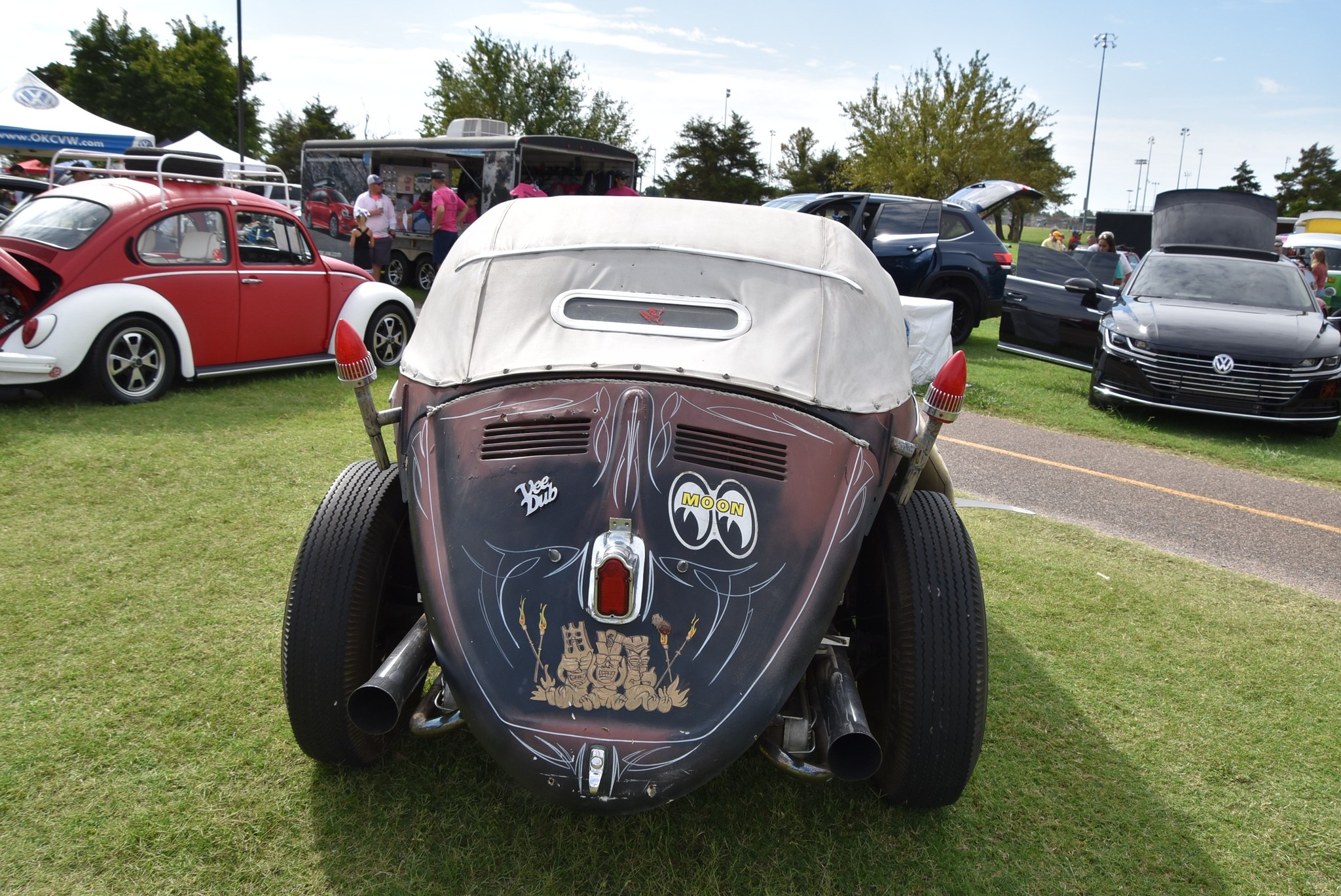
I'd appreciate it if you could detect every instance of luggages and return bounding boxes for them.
[124,147,224,179]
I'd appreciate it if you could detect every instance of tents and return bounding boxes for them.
[153,130,267,198]
[0,68,155,160]
[4,159,50,175]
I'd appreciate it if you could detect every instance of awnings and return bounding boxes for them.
[344,147,484,190]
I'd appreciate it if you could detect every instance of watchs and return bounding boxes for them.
[389,232,396,238]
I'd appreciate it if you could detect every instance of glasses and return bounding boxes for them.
[617,177,628,181]
[1087,240,1090,243]
[375,182,382,185]
[1098,234,1110,239]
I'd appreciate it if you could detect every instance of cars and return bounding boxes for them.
[240,182,302,216]
[304,178,362,238]
[997,189,1340,441]
[0,178,419,406]
[280,197,990,820]
[757,180,1047,346]
[0,174,65,230]
[1273,210,1341,312]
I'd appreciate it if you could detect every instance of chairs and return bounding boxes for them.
[172,231,226,263]
[137,229,169,264]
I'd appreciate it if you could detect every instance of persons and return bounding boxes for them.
[10,165,35,199]
[1086,235,1100,252]
[431,169,469,270]
[1041,228,1081,252]
[401,191,432,233]
[459,190,478,233]
[1310,248,1328,298]
[71,162,95,182]
[349,208,374,275]
[353,174,397,282]
[604,170,641,196]
[1098,231,1133,295]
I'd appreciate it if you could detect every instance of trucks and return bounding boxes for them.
[299,118,641,293]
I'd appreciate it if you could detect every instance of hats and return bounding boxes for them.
[66,161,86,176]
[367,174,386,185]
[614,169,630,178]
[1051,228,1061,240]
[430,170,446,179]
[1072,230,1080,235]
[1274,238,1283,247]
